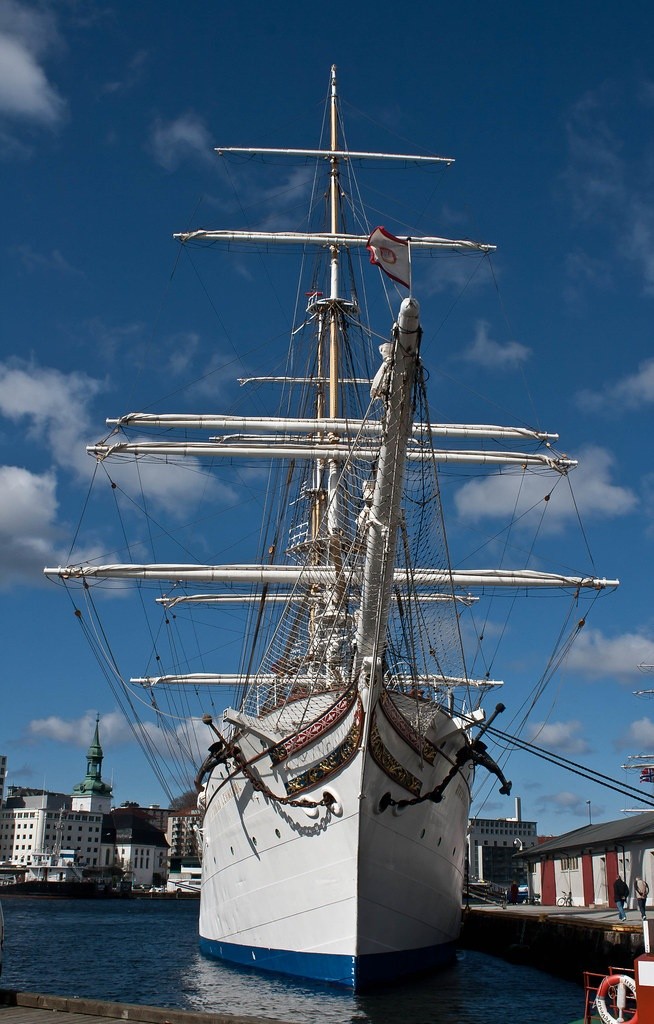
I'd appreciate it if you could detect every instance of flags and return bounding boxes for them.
[366,225,410,289]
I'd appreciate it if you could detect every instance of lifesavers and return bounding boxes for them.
[595,974,638,1024]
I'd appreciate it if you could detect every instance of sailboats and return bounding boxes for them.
[41,61,623,1005]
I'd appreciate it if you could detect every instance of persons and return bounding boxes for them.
[634,875,649,920]
[141,884,145,893]
[511,880,518,905]
[614,875,629,921]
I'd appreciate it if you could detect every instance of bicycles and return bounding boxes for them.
[558,890,572,906]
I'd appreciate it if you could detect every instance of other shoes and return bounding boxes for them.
[642,914,646,920]
[621,916,627,921]
